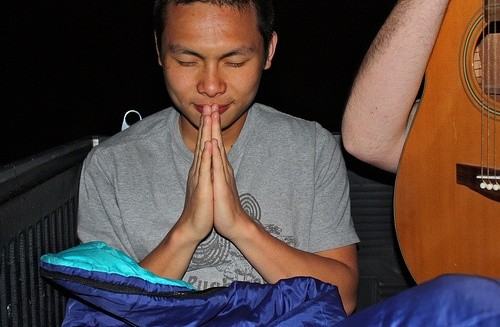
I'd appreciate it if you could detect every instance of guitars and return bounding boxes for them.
[393,0,500,286]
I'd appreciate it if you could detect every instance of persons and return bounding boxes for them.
[76,0,361,317]
[342,1,482,174]
